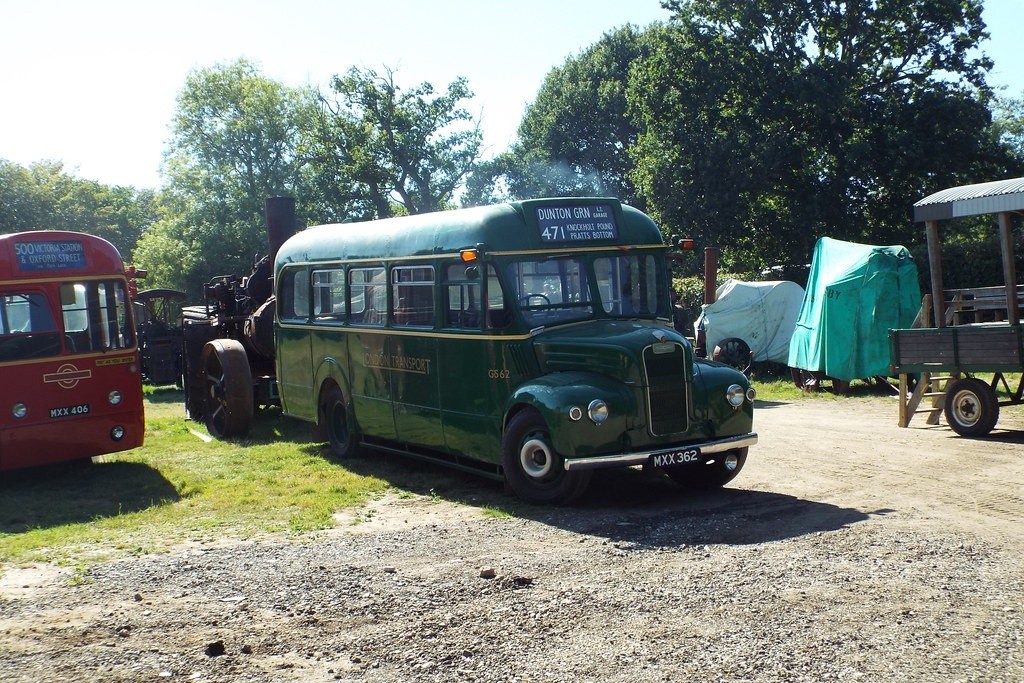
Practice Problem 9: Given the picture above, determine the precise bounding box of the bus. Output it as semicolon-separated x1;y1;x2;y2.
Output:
0;230;148;472
269;196;761;510
